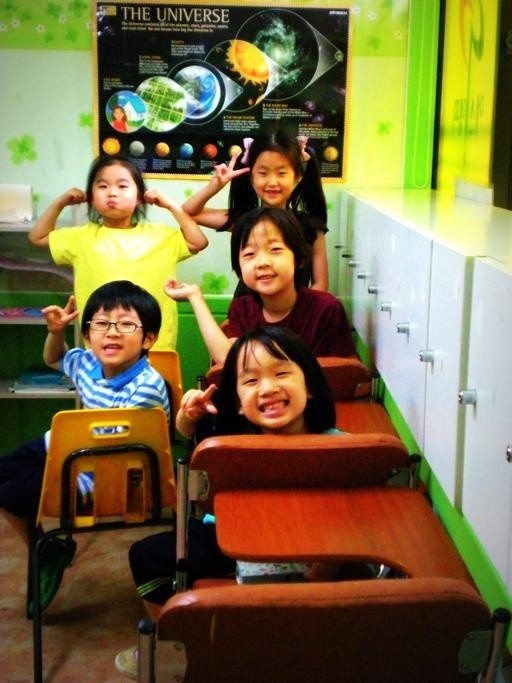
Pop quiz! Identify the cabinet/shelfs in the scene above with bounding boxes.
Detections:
[0,216,83,461]
[336,184,510,592]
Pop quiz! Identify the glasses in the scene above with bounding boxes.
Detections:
[83,319,142,333]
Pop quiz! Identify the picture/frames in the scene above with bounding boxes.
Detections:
[90,0,355,183]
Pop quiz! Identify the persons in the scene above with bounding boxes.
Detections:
[29,154,210,353]
[163,206,358,366]
[181,132,330,299]
[112,322,355,679]
[1,279,173,614]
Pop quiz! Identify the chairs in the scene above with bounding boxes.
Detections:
[26,405,174,681]
[138,484,512,683]
[173,434,422,592]
[194,358,383,406]
[74,347,181,460]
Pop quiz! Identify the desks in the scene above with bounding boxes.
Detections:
[332,395,402,438]
[212,483,478,590]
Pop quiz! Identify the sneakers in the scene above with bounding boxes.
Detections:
[115,645,138,676]
[26,538,76,617]
